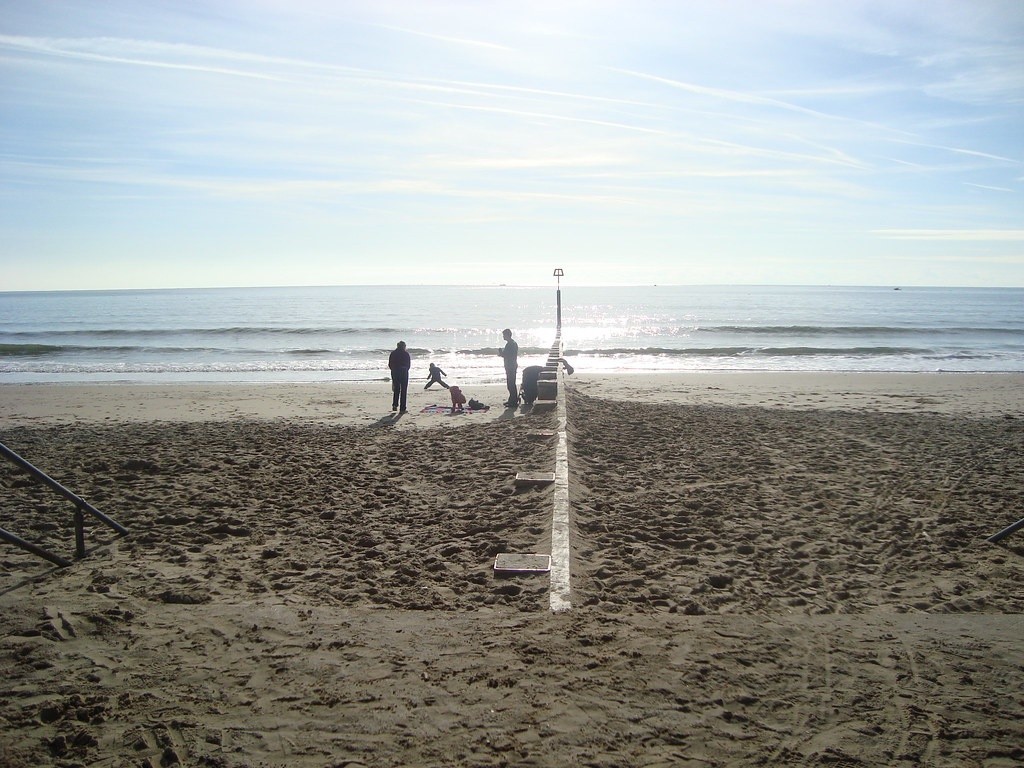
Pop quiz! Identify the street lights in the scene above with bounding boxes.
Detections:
[553,268,564,338]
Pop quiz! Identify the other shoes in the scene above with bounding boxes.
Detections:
[392,407,397,411]
[399,410,408,414]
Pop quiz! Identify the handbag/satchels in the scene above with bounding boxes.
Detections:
[468,399,484,410]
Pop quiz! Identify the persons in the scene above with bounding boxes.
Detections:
[388,341,410,413]
[499,329,519,407]
[424,363,450,390]
[450,386,466,412]
